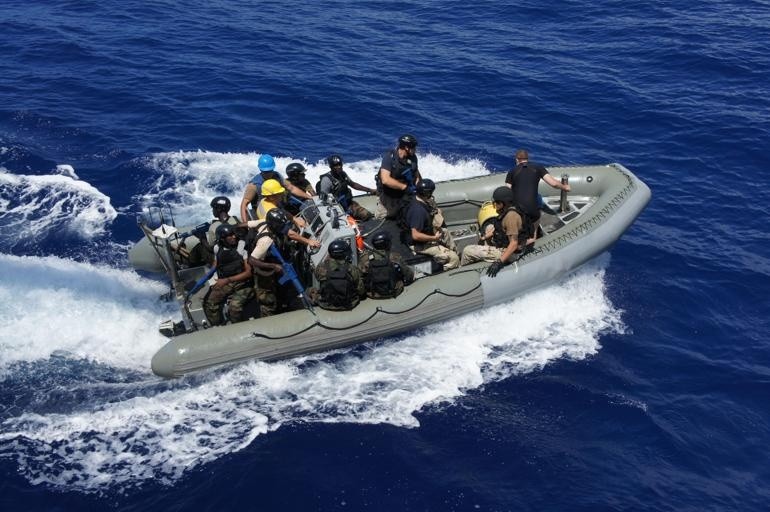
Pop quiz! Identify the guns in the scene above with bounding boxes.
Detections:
[167,220,217,242]
[270,244,317,316]
[284,192,306,210]
[183,266,219,302]
[403,168,418,191]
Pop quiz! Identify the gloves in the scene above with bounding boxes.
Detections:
[486,259,504,277]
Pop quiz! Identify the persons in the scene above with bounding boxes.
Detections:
[502,150,572,230]
[378,134,423,221]
[202,154,413,326]
[403,179,461,272]
[460,185,536,278]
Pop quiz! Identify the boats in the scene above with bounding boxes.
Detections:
[128,164,651,379]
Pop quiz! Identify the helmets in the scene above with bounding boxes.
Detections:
[216,224,235,239]
[399,134,417,148]
[492,186,514,215]
[258,154,307,237]
[328,240,351,259]
[328,155,343,167]
[416,178,435,196]
[211,196,231,212]
[371,231,393,250]
